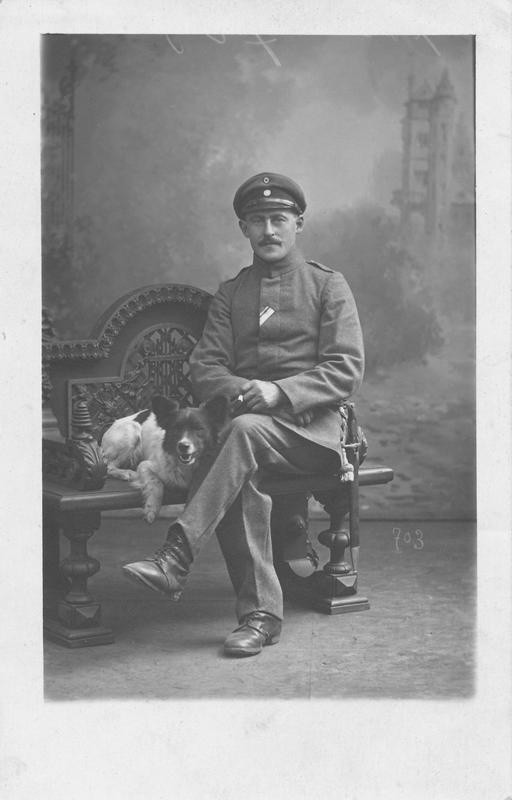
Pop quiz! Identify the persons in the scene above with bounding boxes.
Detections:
[121,172,366,654]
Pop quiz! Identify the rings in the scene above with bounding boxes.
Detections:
[237,394,244,404]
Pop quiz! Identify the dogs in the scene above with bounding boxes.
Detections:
[93,389,230,524]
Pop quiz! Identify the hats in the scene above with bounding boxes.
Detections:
[233,172,306,219]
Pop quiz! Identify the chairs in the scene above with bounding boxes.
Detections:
[42,283,394,648]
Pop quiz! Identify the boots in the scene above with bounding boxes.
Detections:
[120,527,192,603]
[224,611,282,656]
